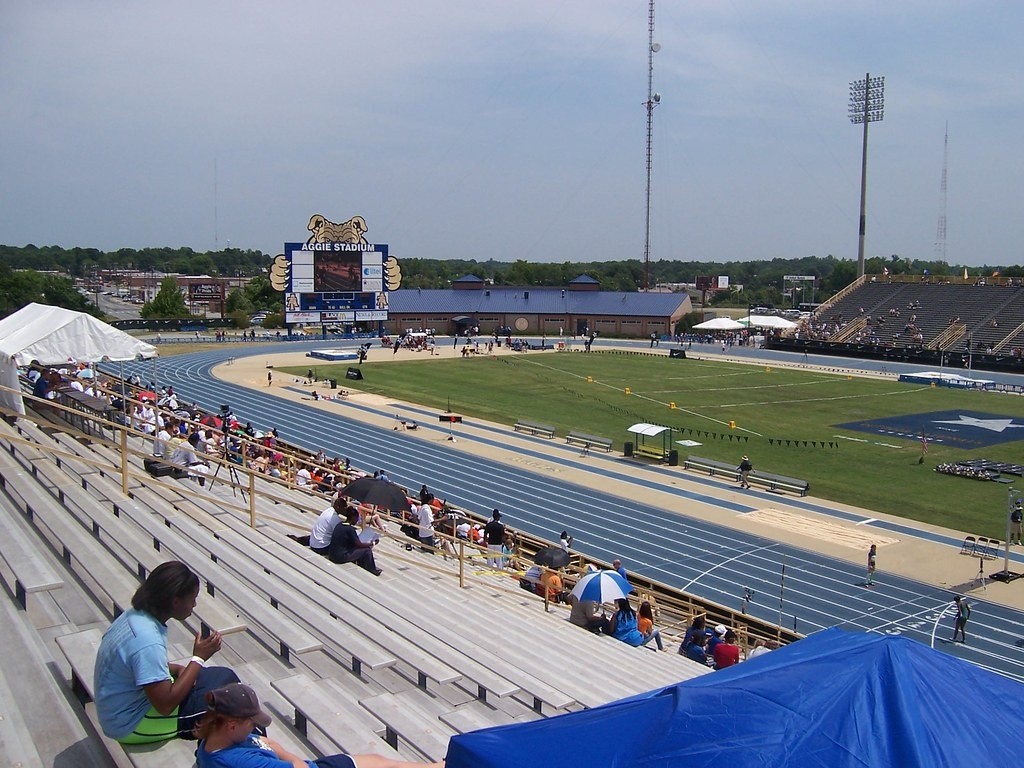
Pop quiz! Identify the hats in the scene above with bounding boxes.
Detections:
[715,624,726,634]
[742,456,749,460]
[189,433,201,441]
[492,509,502,518]
[207,683,272,727]
[1015,504,1023,510]
[272,453,286,463]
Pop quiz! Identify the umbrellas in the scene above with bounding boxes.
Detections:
[536,547,570,568]
[341,474,409,527]
[568,570,632,605]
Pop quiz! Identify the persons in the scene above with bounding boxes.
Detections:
[194,683,445,768]
[1010,506,1024,546]
[542,326,592,350]
[936,315,1024,368]
[794,301,924,345]
[195,329,320,343]
[524,530,779,671]
[27,358,527,576]
[351,326,435,357]
[675,327,788,354]
[308,368,314,386]
[94,561,267,744]
[463,323,528,357]
[869,275,1022,287]
[865,544,876,588]
[650,331,658,348]
[267,370,272,387]
[407,419,421,431]
[949,596,970,643]
[737,455,755,489]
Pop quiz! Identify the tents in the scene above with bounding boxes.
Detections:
[0,302,159,453]
[692,316,797,329]
[448,626,1024,768]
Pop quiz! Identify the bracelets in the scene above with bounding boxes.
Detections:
[189,655,206,667]
[371,538,377,545]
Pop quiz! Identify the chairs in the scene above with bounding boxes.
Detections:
[984,540,1000,561]
[972,537,988,559]
[960,536,977,556]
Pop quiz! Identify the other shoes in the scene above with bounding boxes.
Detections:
[1010,540,1015,546]
[1018,540,1022,546]
[373,569,382,575]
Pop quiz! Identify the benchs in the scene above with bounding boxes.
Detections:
[740,468,810,497]
[0,362,748,768]
